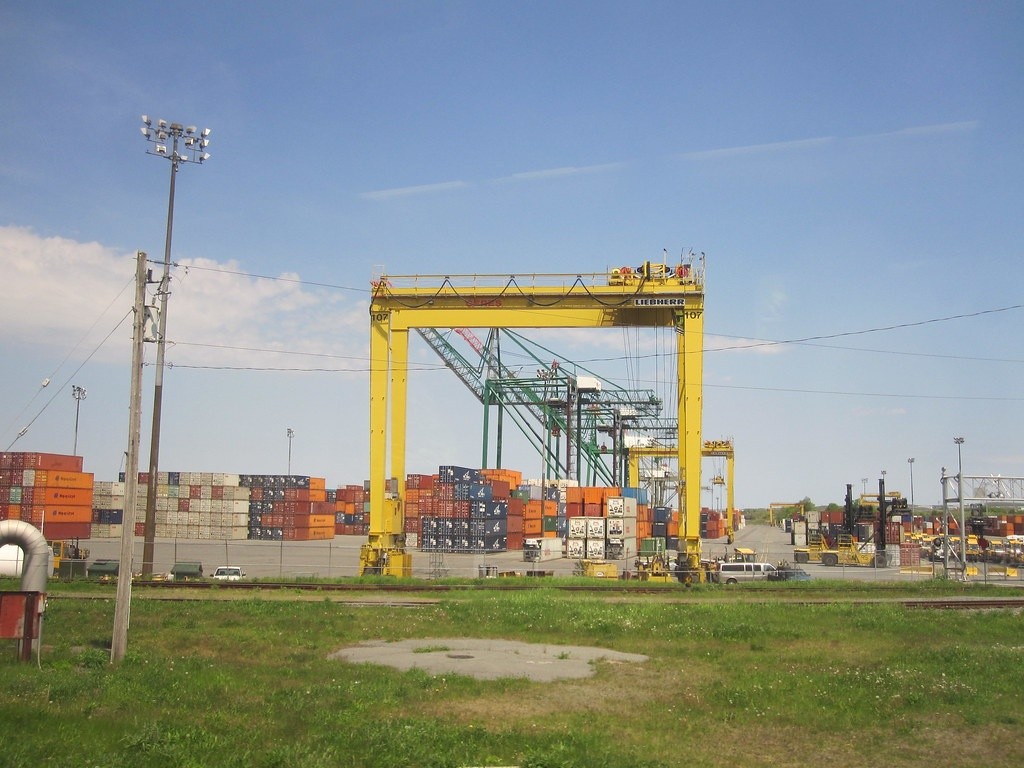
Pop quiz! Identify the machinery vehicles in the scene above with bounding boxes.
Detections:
[369,273,679,505]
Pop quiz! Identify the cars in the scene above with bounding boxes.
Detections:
[207,566,247,583]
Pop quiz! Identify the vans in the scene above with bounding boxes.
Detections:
[716,561,780,585]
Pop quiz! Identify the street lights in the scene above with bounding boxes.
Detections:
[285,428,295,476]
[907,458,916,533]
[881,470,886,480]
[71,383,88,456]
[953,436,966,473]
[140,112,212,578]
[861,477,869,501]
